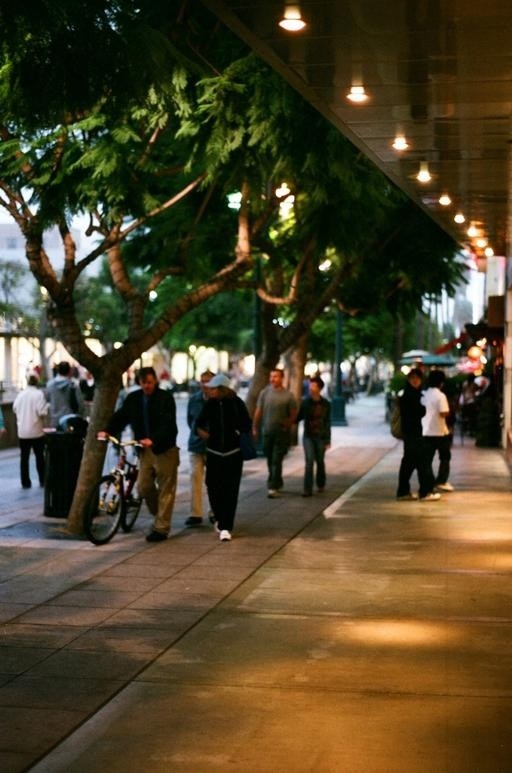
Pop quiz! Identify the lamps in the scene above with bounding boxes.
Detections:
[276,0,308,32]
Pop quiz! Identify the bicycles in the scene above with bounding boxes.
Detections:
[82,432,147,544]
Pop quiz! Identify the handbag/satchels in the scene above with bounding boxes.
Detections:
[391,407,402,438]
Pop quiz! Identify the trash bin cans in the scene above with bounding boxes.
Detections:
[43,433,85,518]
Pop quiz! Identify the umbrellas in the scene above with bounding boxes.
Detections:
[395,353,456,369]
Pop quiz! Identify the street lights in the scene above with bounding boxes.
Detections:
[320,255,353,425]
[229,183,297,455]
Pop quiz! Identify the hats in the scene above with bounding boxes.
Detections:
[205,374,231,387]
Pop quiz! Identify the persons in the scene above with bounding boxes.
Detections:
[291,373,332,496]
[11,372,50,488]
[44,361,85,430]
[461,372,483,439]
[96,366,180,542]
[184,369,215,526]
[251,367,297,497]
[392,367,441,501]
[54,360,176,413]
[418,367,457,491]
[192,373,254,542]
